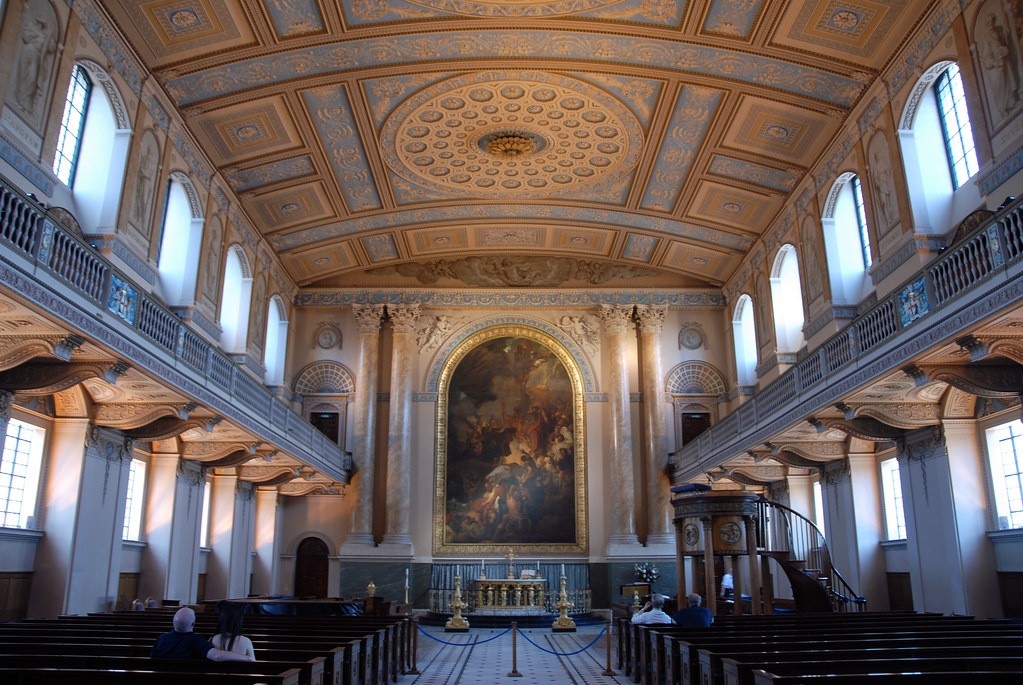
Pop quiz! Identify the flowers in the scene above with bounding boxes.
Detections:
[634,562,659,585]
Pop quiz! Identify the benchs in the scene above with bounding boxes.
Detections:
[616,608,1022,684]
[0,608,415,685]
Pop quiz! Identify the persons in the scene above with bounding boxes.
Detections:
[150,607,255,662]
[721,568,735,613]
[207,606,256,660]
[631,593,672,625]
[670,593,715,627]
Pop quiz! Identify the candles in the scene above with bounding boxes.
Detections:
[405,569,408,587]
[456,565,460,576]
[537,561,540,570]
[561,564,564,577]
[482,560,485,569]
